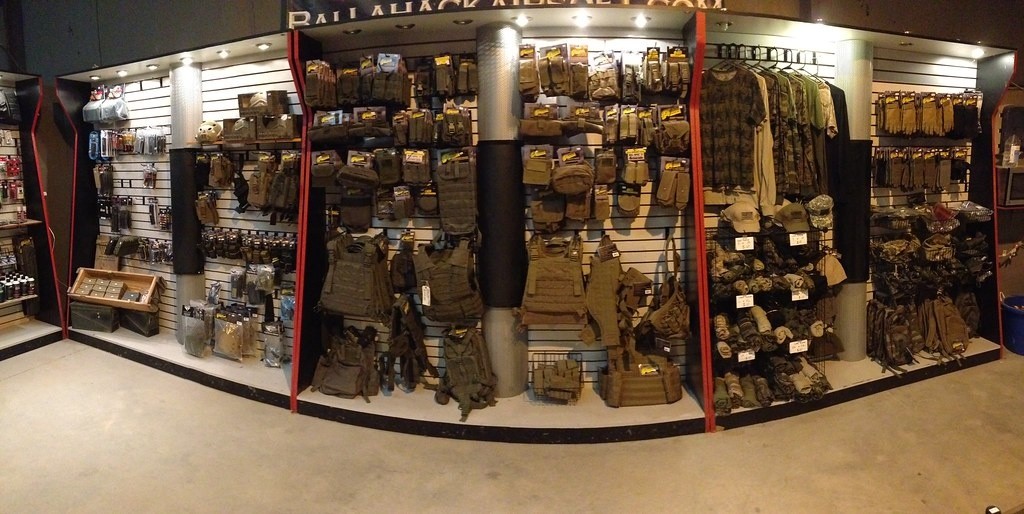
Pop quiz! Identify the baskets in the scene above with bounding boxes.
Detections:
[531,352,582,403]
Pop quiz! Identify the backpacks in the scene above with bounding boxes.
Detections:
[311,326,380,399]
[866,289,980,368]
[441,327,497,413]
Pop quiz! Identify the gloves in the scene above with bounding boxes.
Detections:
[872,88,983,193]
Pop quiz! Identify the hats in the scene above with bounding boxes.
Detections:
[805,195,834,229]
[723,200,761,233]
[774,203,809,233]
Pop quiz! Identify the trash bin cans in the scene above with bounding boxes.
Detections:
[1001,295,1024,356]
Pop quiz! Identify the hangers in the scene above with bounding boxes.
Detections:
[705,42,824,81]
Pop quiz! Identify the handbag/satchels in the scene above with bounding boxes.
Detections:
[551,163,595,195]
[531,199,565,236]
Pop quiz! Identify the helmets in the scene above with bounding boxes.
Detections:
[231,118,250,138]
[249,92,267,114]
[195,121,222,144]
[267,118,287,137]
